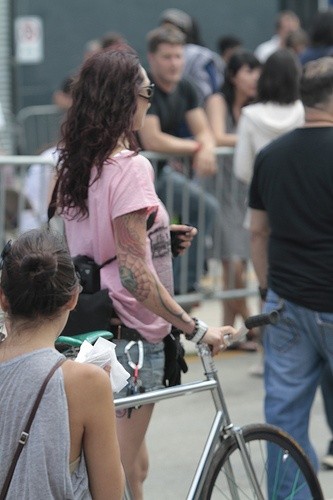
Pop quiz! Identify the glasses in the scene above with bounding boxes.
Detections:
[136,83,156,102]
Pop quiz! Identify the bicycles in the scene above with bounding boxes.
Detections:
[56,311,325,500]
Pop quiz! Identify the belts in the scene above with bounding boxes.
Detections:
[107,323,143,342]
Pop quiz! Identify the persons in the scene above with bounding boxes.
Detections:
[51,7,333,352]
[55,45,237,500]
[247,57,333,500]
[0,230,125,500]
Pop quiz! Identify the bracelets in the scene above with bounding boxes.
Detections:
[259,286,267,301]
[185,318,209,345]
